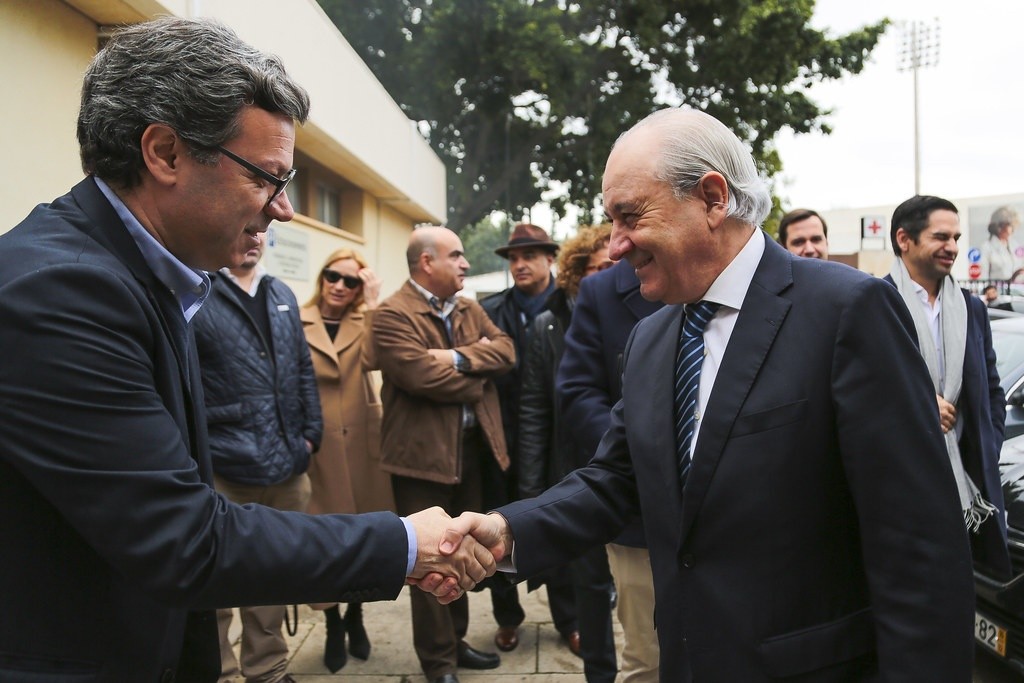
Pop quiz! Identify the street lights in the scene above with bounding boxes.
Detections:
[896,14,940,198]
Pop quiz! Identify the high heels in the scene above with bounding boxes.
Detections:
[322,607,348,675]
[343,607,372,662]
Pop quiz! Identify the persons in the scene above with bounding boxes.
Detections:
[882,196,1010,560]
[479,224,581,653]
[517,223,669,683]
[780,209,830,259]
[195,233,324,683]
[405,108,974,683]
[983,286,998,295]
[298,250,398,672]
[0,18,496,683]
[372,227,515,683]
[981,206,1018,277]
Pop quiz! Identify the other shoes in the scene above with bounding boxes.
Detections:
[611,590,618,609]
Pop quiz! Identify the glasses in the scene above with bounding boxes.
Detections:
[204,141,296,206]
[322,267,363,289]
[580,261,615,274]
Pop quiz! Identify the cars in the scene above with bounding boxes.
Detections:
[971,293,1024,679]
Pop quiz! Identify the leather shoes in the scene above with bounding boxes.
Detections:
[456,643,500,670]
[495,624,518,652]
[434,672,459,683]
[567,629,584,656]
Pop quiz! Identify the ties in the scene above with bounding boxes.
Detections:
[676,300,721,499]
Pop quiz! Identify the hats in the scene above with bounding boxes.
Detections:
[495,223,559,260]
